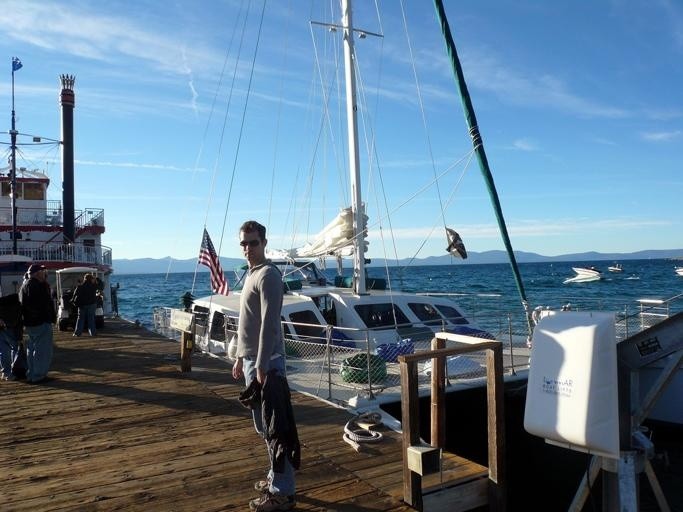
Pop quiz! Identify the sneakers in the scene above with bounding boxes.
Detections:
[32,376,55,385]
[253,479,271,492]
[249,493,296,510]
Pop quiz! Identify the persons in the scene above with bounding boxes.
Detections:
[19,261,55,383]
[95,277,104,292]
[0,292,26,380]
[72,274,104,337]
[230,220,303,512]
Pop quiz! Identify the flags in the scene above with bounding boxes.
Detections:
[197,227,228,296]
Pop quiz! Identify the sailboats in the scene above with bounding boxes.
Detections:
[150,1,557,478]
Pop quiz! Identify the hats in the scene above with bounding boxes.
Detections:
[27,264,46,273]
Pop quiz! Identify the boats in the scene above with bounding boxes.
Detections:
[525,293,681,489]
[571,265,601,276]
[673,267,683,275]
[608,265,623,271]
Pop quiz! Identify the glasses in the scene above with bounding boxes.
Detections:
[240,240,260,246]
